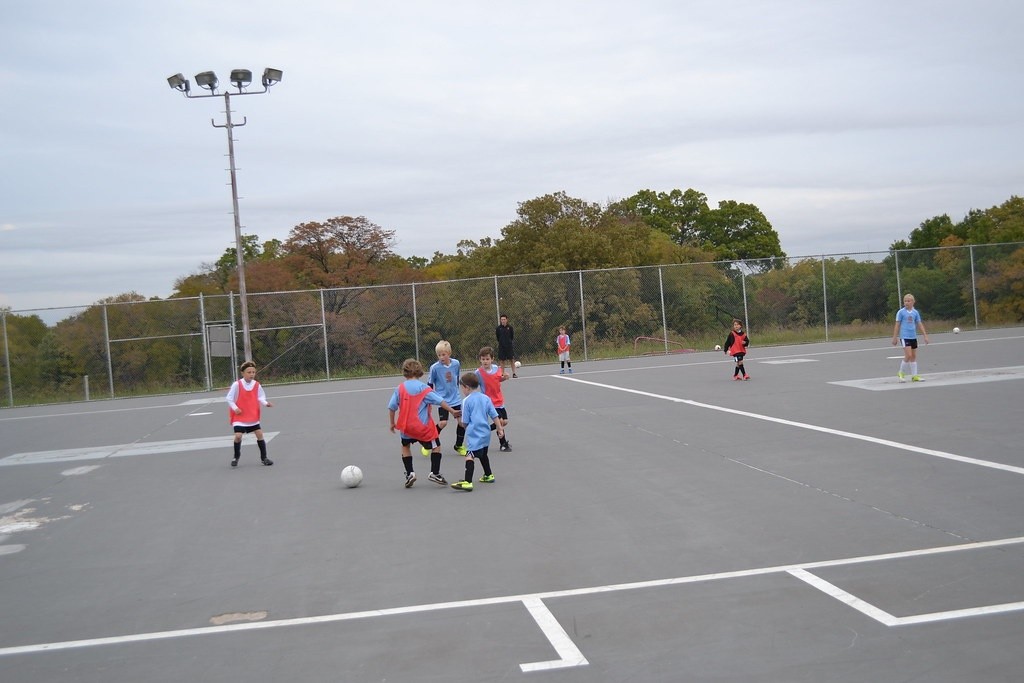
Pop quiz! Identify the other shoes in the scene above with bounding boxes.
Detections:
[569,369,572,374]
[733,375,742,380]
[560,370,565,374]
[743,374,750,380]
[513,374,517,378]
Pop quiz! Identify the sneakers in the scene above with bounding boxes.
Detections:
[500,441,512,452]
[422,446,430,457]
[479,474,494,482]
[912,375,925,382]
[405,472,416,488]
[231,457,239,466]
[897,371,905,383]
[454,445,467,456]
[262,458,273,465]
[451,480,473,492]
[428,471,448,485]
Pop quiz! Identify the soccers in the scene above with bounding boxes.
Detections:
[515,361,521,368]
[953,327,959,334]
[340,465,363,488]
[715,345,721,351]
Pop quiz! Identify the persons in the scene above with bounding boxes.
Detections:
[892,294,928,381]
[451,372,503,492]
[474,347,511,452]
[388,359,460,488]
[496,315,517,378]
[421,340,467,457]
[724,319,750,380]
[557,326,573,374]
[227,361,273,465]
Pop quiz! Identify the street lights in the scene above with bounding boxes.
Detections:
[167,68,283,363]
